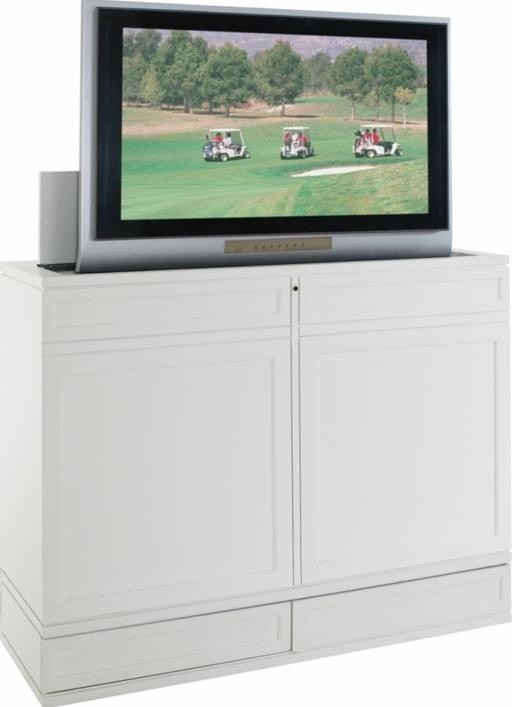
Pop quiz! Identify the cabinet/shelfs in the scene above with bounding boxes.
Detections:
[0,251,512,707]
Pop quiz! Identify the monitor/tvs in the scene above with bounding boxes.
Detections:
[77,0,451,269]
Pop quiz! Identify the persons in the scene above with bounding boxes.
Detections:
[213,133,237,152]
[284,132,308,146]
[362,128,379,145]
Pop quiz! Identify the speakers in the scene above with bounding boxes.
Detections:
[35,170,79,268]
